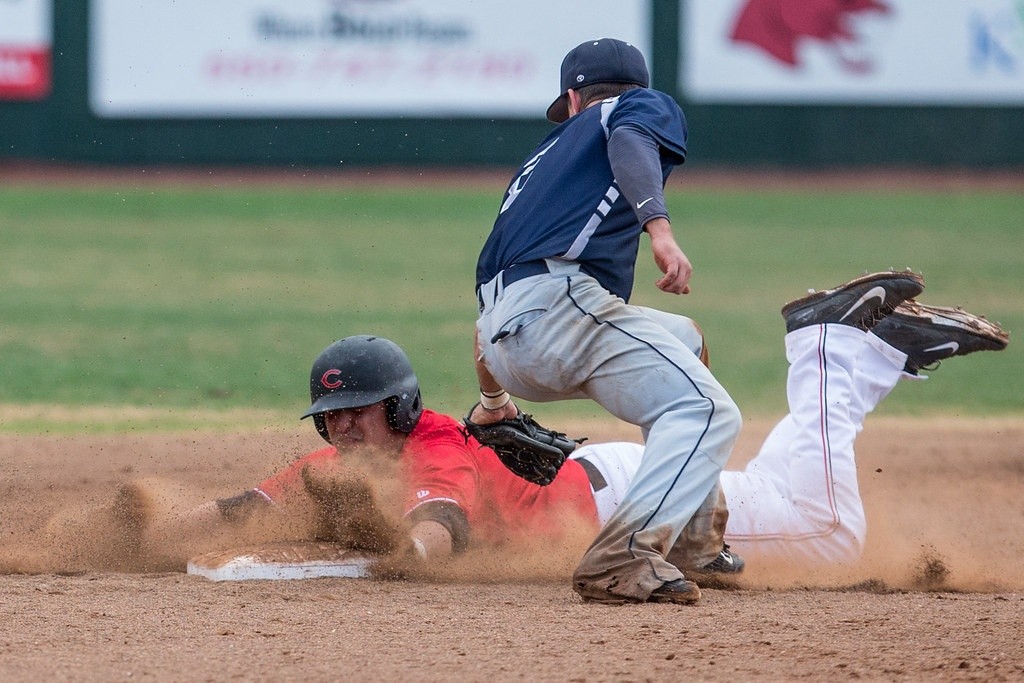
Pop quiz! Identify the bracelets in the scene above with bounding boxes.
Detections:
[478,388,511,411]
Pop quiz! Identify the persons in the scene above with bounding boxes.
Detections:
[140,269,1010,576]
[467,39,746,606]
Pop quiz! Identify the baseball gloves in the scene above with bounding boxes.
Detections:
[463,399,577,488]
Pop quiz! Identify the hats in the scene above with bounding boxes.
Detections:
[546,38,649,124]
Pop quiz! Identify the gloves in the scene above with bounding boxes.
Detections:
[491,325,521,344]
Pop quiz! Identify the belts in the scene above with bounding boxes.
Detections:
[479,258,594,307]
[574,457,609,493]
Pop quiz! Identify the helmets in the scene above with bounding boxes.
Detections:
[300,335,423,447]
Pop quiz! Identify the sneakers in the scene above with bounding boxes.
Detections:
[573,571,701,605]
[781,265,925,337]
[869,299,1010,376]
[693,540,746,576]
